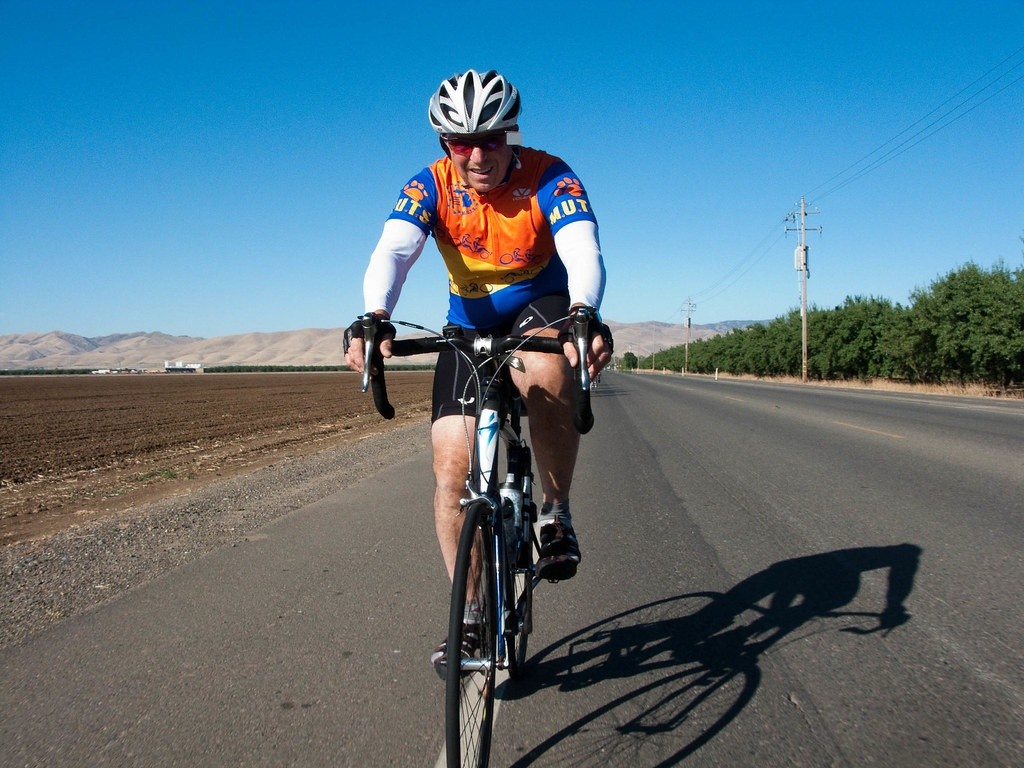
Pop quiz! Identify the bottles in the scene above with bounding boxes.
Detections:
[499,473,522,548]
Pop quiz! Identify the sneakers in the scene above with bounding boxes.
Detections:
[535,513,581,579]
[430,622,480,680]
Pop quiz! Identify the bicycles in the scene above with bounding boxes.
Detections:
[359,308,595,767]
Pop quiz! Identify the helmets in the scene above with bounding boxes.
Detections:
[427,68,521,134]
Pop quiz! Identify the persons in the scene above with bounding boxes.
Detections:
[342,69,615,681]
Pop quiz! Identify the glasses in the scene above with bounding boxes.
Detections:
[441,127,515,156]
